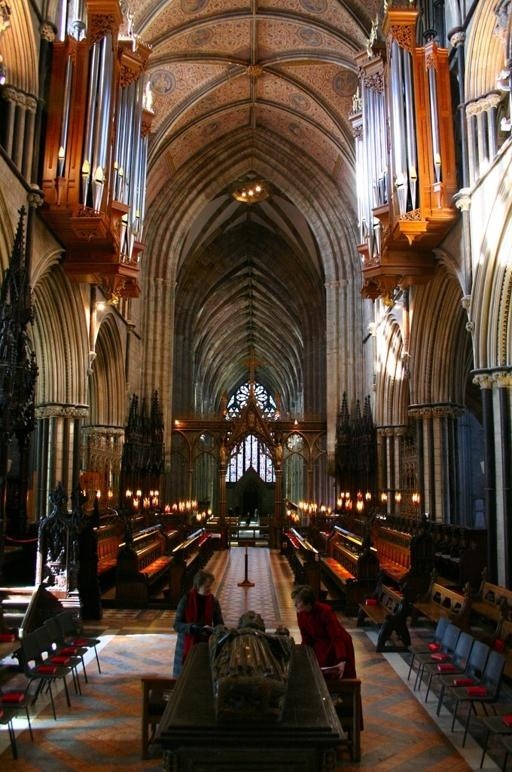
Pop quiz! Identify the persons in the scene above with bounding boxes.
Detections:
[173,570,226,680]
[290,583,365,735]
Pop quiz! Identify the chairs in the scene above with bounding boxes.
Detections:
[0,608,102,762]
[405,615,512,770]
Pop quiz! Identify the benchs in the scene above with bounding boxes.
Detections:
[78,503,227,620]
[280,490,510,658]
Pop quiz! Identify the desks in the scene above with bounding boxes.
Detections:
[138,672,363,764]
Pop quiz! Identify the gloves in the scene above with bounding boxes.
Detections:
[189,624,205,636]
